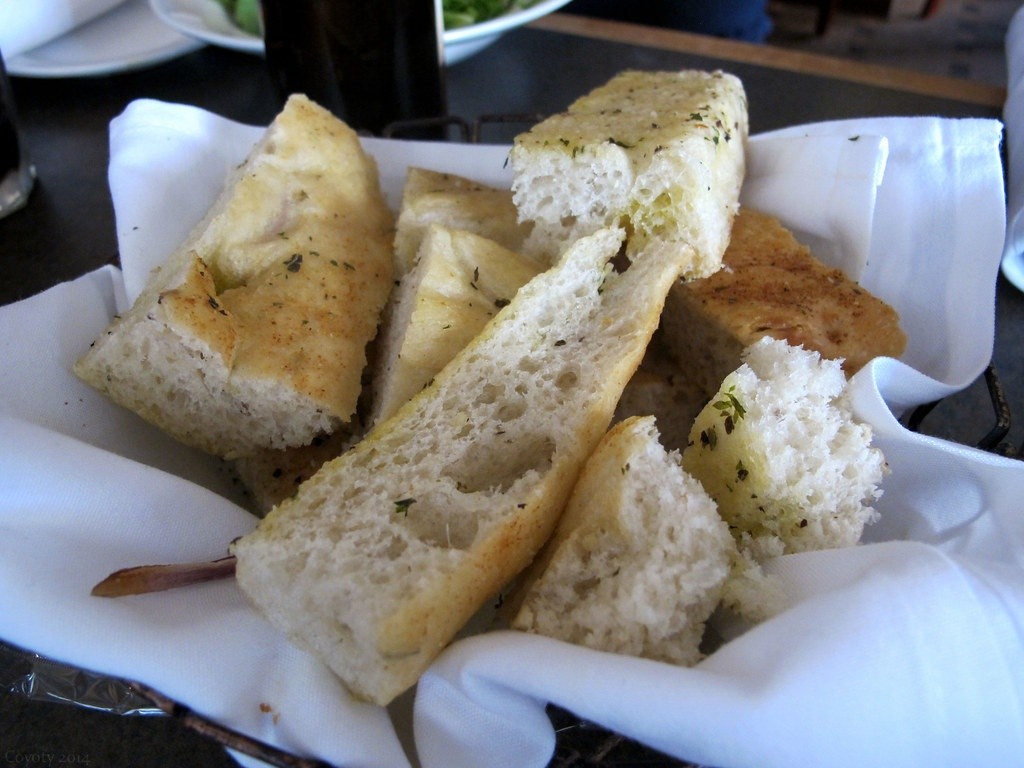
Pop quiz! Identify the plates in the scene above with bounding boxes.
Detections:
[5,1,207,77]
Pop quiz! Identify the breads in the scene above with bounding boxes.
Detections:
[71,71,908,707]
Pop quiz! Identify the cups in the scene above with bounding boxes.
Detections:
[0,53,35,218]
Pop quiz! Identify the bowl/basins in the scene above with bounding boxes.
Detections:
[147,2,573,68]
